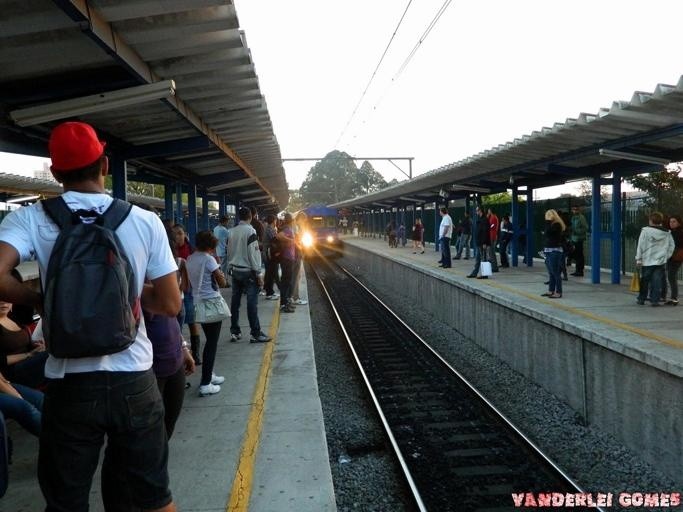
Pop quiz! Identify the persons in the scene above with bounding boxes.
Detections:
[352,218,359,237]
[569,204,588,276]
[635,213,676,306]
[338,216,348,235]
[637,263,668,301]
[664,215,683,306]
[387,205,512,279]
[540,209,568,298]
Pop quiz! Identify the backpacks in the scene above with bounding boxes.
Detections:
[39,195,141,359]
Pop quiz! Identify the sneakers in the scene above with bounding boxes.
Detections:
[258,290,280,300]
[636,298,679,306]
[250,332,271,342]
[541,291,562,298]
[230,333,241,342]
[280,289,307,313]
[200,383,220,395]
[212,375,224,384]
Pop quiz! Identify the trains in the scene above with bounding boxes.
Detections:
[291,206,340,260]
[0,170,234,229]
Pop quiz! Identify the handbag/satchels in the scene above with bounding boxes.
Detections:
[195,294,232,324]
[631,272,640,291]
[481,262,493,276]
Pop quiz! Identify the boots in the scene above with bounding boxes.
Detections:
[190,335,202,365]
